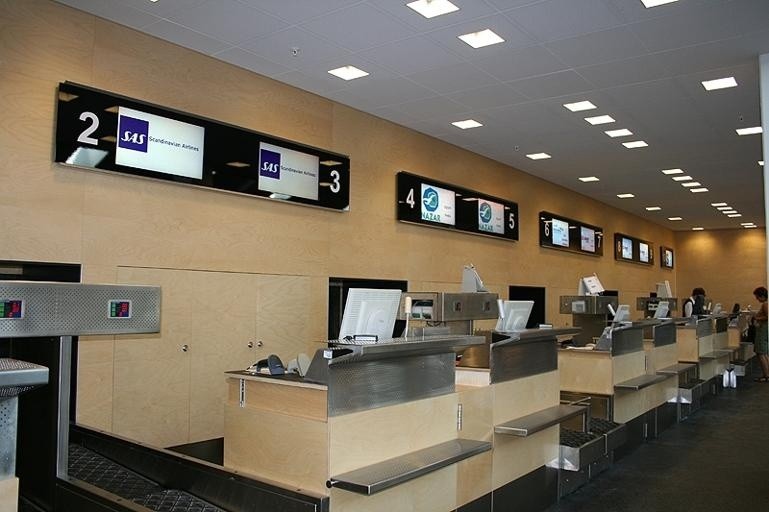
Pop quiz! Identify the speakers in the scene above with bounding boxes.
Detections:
[268,354,284,375]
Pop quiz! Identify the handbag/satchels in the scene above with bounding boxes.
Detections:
[747,316,756,344]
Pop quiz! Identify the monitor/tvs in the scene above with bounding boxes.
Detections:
[712,303,722,315]
[692,295,704,314]
[496,300,535,335]
[653,301,670,319]
[613,305,632,322]
[340,287,402,342]
[730,302,740,321]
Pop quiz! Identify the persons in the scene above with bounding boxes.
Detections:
[753,287,769,382]
[683,288,706,317]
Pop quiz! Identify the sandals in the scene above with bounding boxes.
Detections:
[753,376,769,383]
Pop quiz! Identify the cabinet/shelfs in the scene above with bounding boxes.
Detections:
[224,311,750,512]
